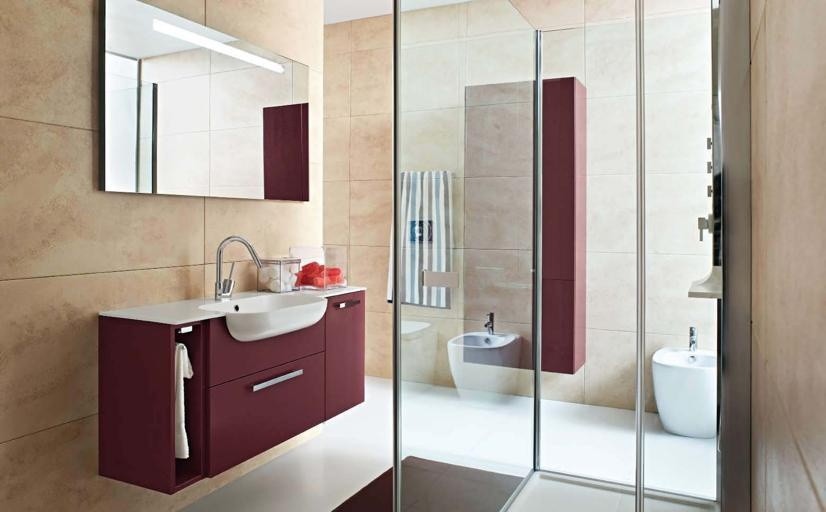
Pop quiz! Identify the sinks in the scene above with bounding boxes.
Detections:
[446,332,523,405]
[197,291,330,342]
[651,346,717,439]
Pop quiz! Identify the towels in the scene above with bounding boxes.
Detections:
[384,169,454,309]
[173,338,196,463]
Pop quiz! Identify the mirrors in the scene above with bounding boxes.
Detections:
[96,0,311,202]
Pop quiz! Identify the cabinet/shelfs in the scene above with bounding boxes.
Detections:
[95,311,207,496]
[324,289,366,424]
[458,73,588,376]
[206,301,325,477]
[260,102,308,203]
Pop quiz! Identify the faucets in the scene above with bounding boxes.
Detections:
[483,312,495,335]
[213,234,263,302]
[688,325,698,351]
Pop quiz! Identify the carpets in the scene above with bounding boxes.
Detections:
[329,455,525,511]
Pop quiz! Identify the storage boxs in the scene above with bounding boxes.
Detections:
[254,258,301,294]
[288,241,348,292]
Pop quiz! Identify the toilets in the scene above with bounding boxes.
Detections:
[401,317,440,398]
